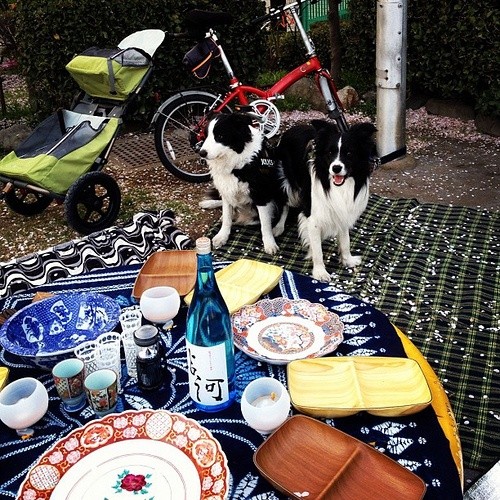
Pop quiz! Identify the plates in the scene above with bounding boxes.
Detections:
[229,297,348,362]
[15,408,232,500]
[184,258,285,314]
[287,356,432,419]
[130,249,198,299]
[252,414,426,500]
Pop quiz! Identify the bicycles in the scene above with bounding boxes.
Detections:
[151,0,350,184]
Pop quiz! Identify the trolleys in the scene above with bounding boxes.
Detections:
[0,28,190,236]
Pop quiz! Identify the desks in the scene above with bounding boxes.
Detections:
[0,262,465,500]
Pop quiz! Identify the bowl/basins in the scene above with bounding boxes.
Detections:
[240,376,291,436]
[0,377,47,430]
[139,286,183,324]
[0,292,121,369]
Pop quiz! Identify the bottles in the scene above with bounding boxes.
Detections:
[133,324,170,398]
[184,237,236,413]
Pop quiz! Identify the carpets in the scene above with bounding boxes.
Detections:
[200,192,500,493]
[0,208,191,296]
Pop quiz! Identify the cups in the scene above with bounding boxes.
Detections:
[51,358,87,412]
[83,369,118,416]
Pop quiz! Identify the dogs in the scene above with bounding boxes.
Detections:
[197,108,288,255]
[276,119,380,281]
